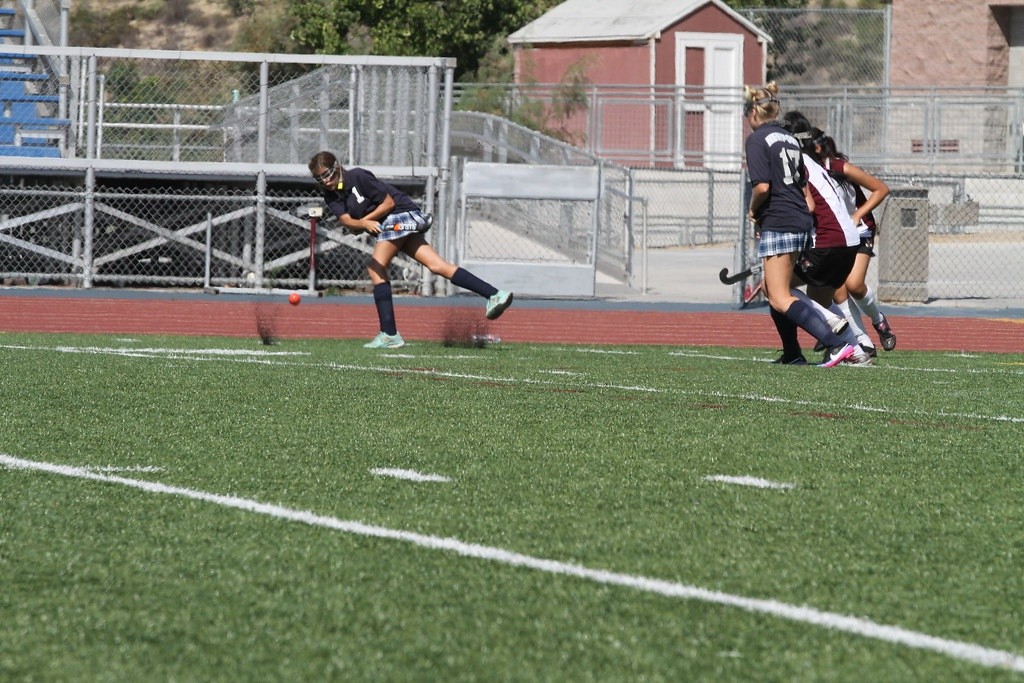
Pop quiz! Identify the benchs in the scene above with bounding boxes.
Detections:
[0,8,71,158]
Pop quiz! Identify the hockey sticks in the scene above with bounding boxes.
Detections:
[720,262,764,285]
[378,213,433,232]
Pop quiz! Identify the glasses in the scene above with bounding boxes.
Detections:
[741,96,780,118]
[311,161,340,186]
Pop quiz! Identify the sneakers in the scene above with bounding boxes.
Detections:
[771,349,807,366]
[363,330,404,347]
[811,316,848,352]
[835,345,873,366]
[858,342,876,358]
[872,311,896,350]
[817,341,855,367]
[485,291,513,320]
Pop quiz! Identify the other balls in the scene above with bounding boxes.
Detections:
[288,293,301,305]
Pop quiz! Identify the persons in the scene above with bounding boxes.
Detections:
[743,81,896,368]
[309,151,513,349]
